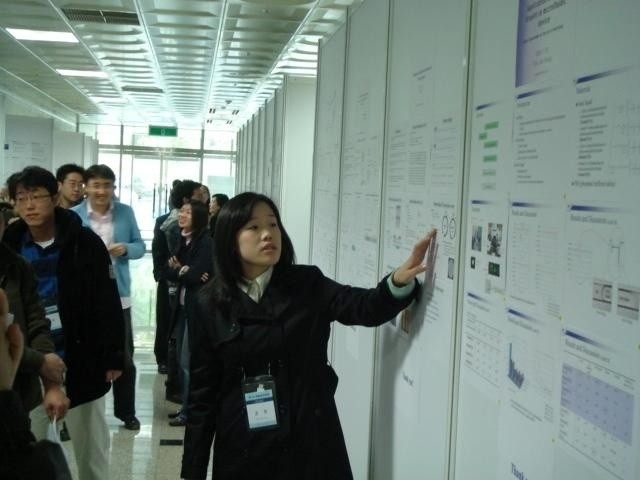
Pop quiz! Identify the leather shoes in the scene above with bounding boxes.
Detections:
[123,415,140,430]
[158,363,168,374]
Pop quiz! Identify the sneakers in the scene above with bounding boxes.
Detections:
[167,408,187,426]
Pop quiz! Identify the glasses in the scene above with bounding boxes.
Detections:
[17,193,51,204]
